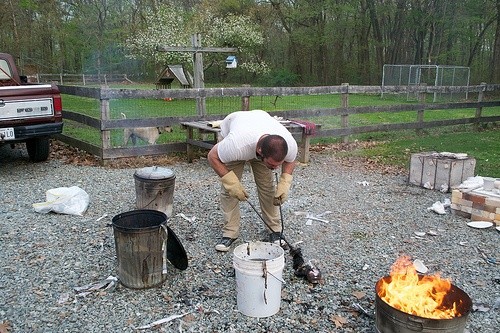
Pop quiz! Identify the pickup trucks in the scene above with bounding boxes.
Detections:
[0,53,64,163]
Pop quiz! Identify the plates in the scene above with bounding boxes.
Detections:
[467,221,493,228]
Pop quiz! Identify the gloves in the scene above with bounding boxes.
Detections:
[220,169,249,202]
[272,172,293,207]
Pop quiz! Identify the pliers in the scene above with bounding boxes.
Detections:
[245,173,292,250]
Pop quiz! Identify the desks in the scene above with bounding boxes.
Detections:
[180,119,322,163]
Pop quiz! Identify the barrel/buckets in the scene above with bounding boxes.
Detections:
[232,240,285,317]
[112,209,169,288]
[377,276,472,333]
[133,167,175,220]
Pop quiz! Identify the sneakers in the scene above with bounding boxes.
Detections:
[270,232,285,246]
[215,237,238,252]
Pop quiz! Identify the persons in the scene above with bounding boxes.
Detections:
[208,110,298,251]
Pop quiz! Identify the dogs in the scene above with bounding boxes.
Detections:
[121,112,173,145]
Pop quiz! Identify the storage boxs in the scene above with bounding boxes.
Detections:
[408,152,476,192]
